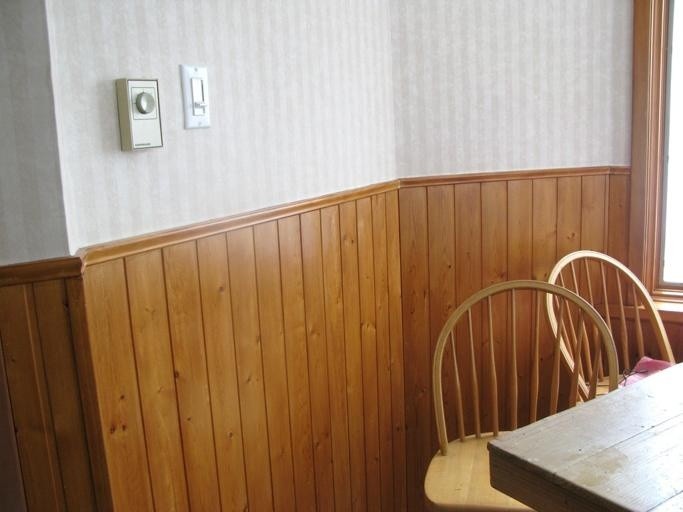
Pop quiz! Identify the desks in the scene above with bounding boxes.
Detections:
[489,360,683,512]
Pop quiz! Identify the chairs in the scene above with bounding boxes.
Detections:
[425,279,619,512]
[543,248,676,404]
